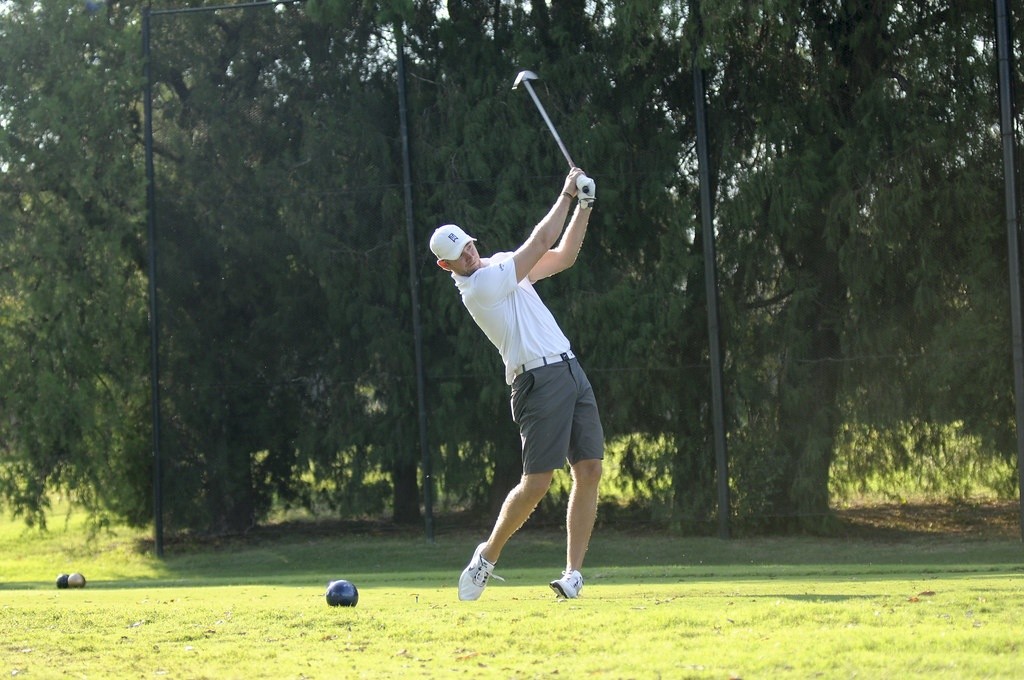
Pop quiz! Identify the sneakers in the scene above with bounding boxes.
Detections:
[549,570,583,599]
[458,542,505,601]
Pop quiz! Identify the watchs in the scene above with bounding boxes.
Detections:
[579,199,594,208]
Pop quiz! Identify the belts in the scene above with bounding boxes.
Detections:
[512,350,575,383]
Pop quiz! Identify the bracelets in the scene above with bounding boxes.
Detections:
[561,192,574,200]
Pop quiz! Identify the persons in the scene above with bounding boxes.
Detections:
[430,168,606,601]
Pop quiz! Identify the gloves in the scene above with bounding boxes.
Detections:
[560,167,586,200]
[577,174,596,207]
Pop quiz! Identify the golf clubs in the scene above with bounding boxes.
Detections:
[512,69,591,194]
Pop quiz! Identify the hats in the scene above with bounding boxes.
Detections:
[429,224,477,260]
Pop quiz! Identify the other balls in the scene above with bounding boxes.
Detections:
[326,579,358,606]
[68,573,85,587]
[56,574,69,588]
